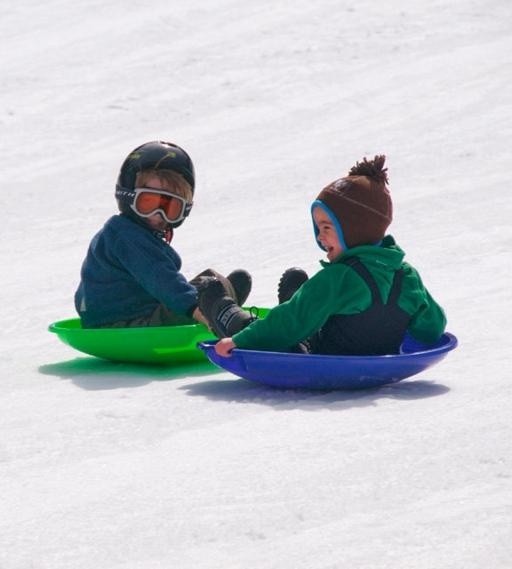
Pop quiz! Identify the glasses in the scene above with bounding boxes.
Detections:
[116,186,194,225]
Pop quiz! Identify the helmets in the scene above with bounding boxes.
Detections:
[117,139,195,227]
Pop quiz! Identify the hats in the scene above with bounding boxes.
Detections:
[311,155,393,250]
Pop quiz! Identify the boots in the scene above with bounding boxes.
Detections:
[198,269,258,336]
[278,267,307,305]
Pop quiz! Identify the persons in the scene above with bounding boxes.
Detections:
[193,151,450,362]
[72,139,253,327]
